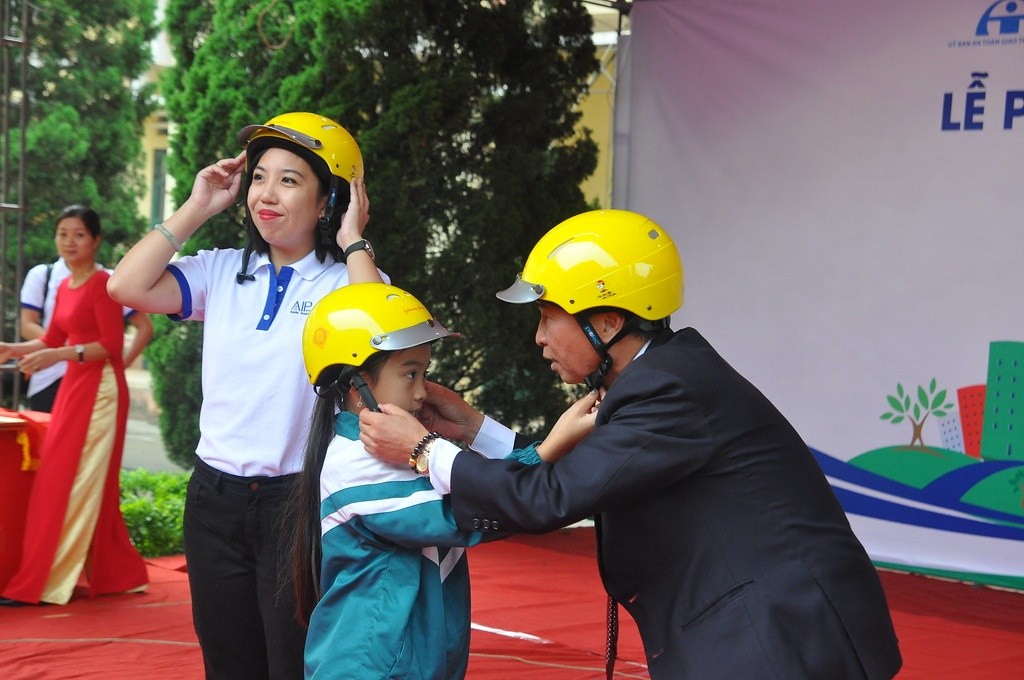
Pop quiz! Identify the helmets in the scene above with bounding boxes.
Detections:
[496,209,683,321]
[239,112,364,207]
[302,282,460,387]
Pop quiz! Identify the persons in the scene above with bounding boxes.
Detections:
[279,284,607,680]
[357,209,902,680]
[106,112,391,680]
[0,204,154,607]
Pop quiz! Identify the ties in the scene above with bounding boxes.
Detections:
[606,596,618,680]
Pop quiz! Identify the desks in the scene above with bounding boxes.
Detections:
[0,408,52,599]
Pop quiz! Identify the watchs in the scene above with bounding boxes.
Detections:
[415,438,441,475]
[343,239,376,265]
[75,344,84,363]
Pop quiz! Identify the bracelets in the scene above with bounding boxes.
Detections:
[154,223,181,252]
[409,431,446,475]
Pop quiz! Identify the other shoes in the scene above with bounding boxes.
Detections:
[0,597,49,606]
